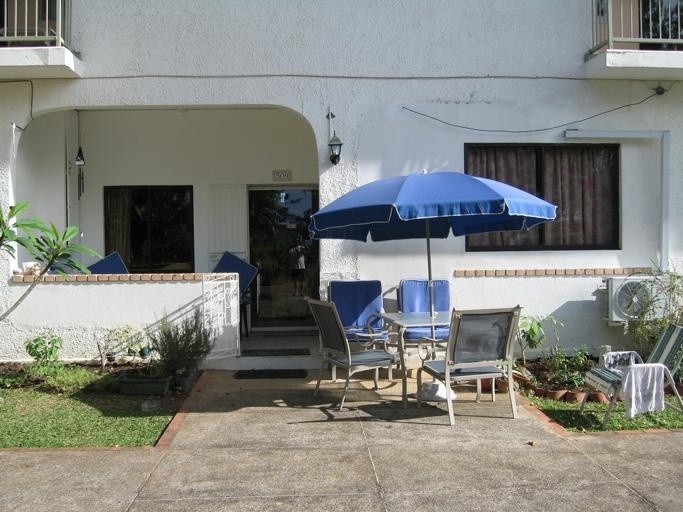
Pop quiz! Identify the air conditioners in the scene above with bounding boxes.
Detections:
[598,274,682,327]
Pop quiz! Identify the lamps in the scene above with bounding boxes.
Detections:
[328,130,345,165]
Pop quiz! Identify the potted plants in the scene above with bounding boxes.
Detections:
[482,314,624,404]
[97,306,217,399]
[621,251,683,397]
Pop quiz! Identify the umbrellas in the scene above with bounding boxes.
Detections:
[308,169,559,382]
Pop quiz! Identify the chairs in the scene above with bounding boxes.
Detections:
[302,278,524,426]
[576,323,682,429]
[240,291,253,341]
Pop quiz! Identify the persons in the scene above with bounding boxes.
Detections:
[286,237,309,296]
